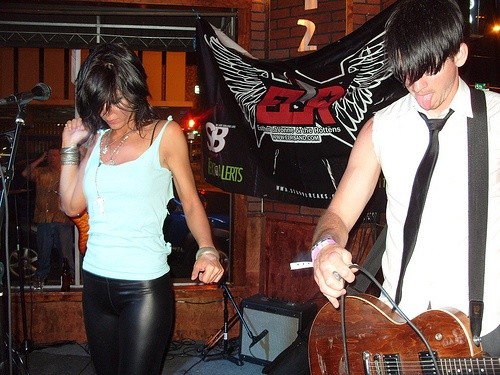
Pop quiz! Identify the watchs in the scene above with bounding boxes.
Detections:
[290,234,335,271]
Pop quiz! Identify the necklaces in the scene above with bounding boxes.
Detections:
[101,128,133,155]
[99,128,135,163]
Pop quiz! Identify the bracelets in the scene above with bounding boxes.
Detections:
[195,247,220,263]
[61,147,80,165]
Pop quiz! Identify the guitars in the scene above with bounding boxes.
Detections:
[308,293,500,375]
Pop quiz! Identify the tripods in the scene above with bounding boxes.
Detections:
[200,192,244,366]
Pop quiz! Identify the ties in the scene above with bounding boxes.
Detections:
[392,108,455,312]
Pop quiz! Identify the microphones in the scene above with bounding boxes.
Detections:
[249,329,269,348]
[0,81,52,106]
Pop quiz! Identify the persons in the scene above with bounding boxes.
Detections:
[58,43,223,375]
[22,148,76,289]
[291,0,500,358]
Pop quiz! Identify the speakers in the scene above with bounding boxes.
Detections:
[238,293,312,364]
